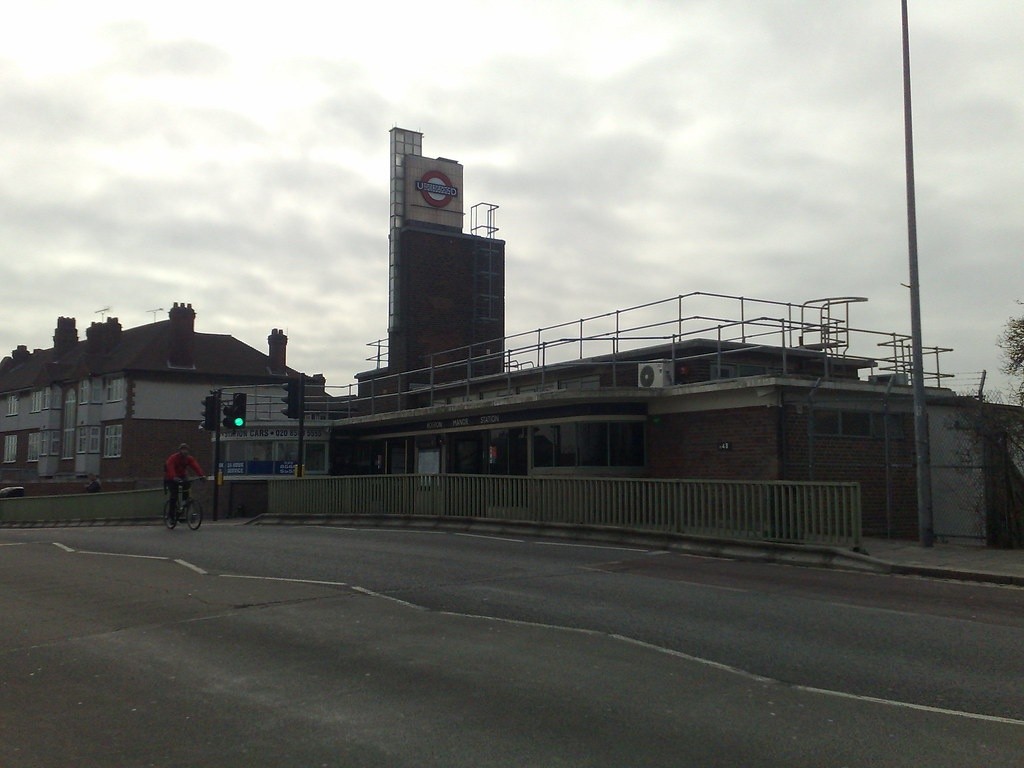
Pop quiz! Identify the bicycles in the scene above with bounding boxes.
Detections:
[164,476,212,530]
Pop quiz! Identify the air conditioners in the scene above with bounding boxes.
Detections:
[637,358,675,388]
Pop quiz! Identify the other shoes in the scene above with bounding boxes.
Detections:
[167,515,174,524]
[181,495,189,500]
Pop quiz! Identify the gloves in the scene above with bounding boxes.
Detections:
[174,476,183,486]
[199,474,207,484]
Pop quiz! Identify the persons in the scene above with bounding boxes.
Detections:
[86,473,100,493]
[165,443,205,525]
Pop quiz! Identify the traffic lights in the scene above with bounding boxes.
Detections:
[281,373,304,419]
[201,389,220,431]
[223,392,246,429]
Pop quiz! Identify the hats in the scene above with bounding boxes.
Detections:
[178,443,191,450]
